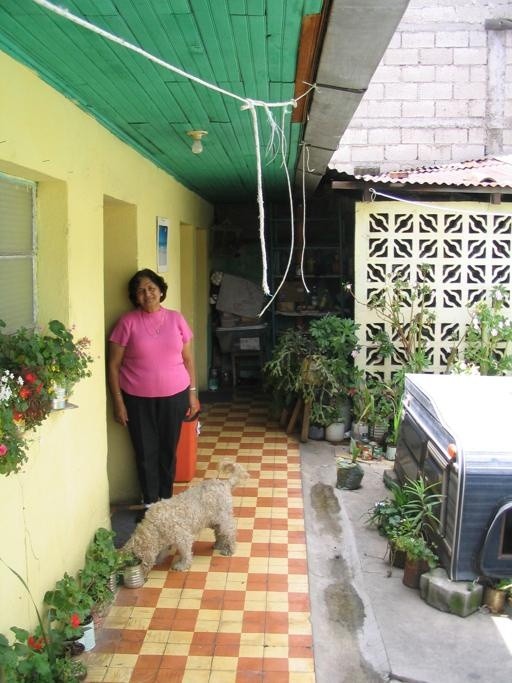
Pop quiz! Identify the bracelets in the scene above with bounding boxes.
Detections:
[187,386,197,391]
[111,390,122,398]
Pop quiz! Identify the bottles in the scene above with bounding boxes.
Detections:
[312,285,329,312]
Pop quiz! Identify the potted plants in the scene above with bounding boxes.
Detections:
[260,312,401,461]
[484,578,512,614]
[358,470,448,591]
[42,528,145,652]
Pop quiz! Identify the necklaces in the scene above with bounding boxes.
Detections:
[141,308,166,336]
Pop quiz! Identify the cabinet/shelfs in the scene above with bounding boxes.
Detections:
[269,196,344,346]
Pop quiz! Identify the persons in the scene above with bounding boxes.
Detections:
[107,267,200,522]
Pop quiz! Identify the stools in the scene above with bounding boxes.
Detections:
[231,351,267,400]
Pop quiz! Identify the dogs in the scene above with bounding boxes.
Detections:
[120,459,248,573]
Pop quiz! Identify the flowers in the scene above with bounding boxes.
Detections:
[0,613,88,682]
[0,317,102,477]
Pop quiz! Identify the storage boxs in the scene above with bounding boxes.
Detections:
[215,322,270,354]
